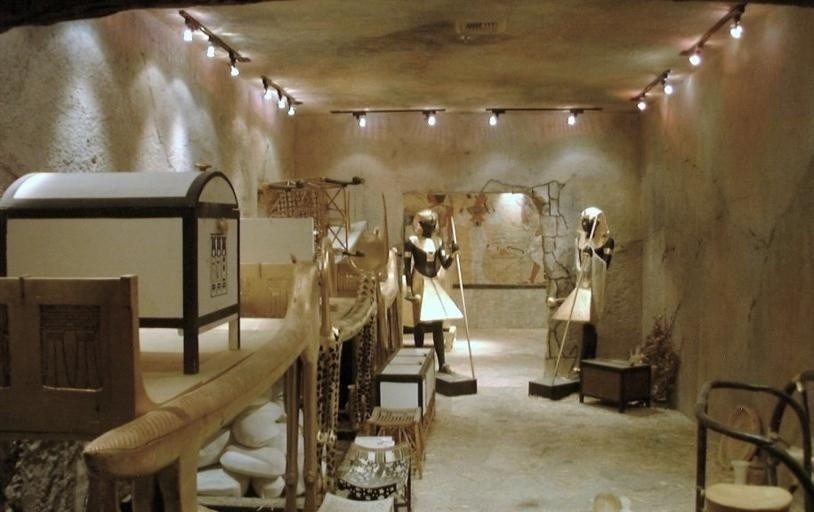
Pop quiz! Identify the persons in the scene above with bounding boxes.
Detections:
[403,208,460,374]
[551,207,615,361]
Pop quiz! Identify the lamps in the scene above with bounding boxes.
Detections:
[634,68,673,112]
[351,110,437,126]
[178,11,252,74]
[488,109,583,125]
[261,76,304,116]
[680,0,744,68]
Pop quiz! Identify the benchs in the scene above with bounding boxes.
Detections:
[580,356,653,414]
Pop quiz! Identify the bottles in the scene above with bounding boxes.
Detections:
[750,455,767,484]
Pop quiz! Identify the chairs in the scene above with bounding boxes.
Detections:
[692,376,814,512]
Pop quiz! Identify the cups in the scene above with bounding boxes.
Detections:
[731,459,748,483]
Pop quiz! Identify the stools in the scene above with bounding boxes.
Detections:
[368,406,427,480]
[335,441,414,512]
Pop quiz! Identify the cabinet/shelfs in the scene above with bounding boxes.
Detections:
[374,345,440,435]
